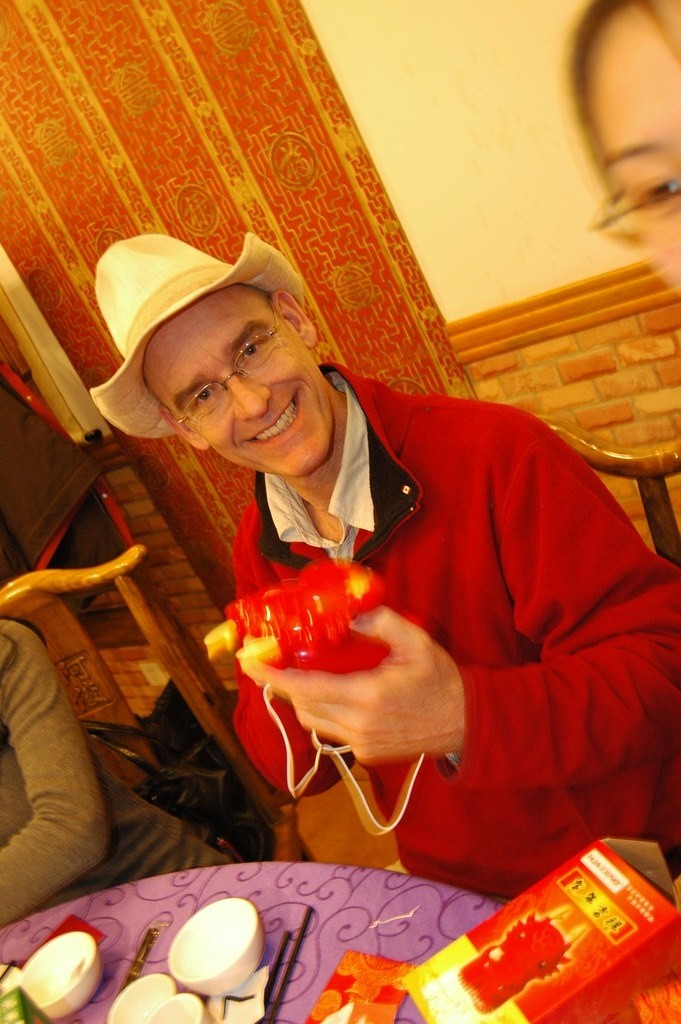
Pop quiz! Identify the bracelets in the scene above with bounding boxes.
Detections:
[441,751,462,767]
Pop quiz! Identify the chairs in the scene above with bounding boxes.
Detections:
[536,416,681,570]
[1,544,302,862]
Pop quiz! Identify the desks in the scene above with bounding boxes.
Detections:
[1,860,509,1024]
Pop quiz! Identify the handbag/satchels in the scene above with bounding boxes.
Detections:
[79,719,272,862]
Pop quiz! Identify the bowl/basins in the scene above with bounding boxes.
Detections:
[16,932,102,1021]
[139,994,216,1022]
[166,897,265,993]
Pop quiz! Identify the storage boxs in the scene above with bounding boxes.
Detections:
[402,838,680,1024]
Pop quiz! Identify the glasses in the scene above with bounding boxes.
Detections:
[170,300,278,424]
[595,187,681,256]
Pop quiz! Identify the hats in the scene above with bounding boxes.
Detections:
[90,231,304,438]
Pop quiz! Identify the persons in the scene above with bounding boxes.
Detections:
[0,617,235,928]
[88,232,681,904]
[569,1,681,306]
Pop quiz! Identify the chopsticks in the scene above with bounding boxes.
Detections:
[253,905,312,1022]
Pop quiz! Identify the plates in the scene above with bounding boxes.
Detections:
[104,973,176,1022]
